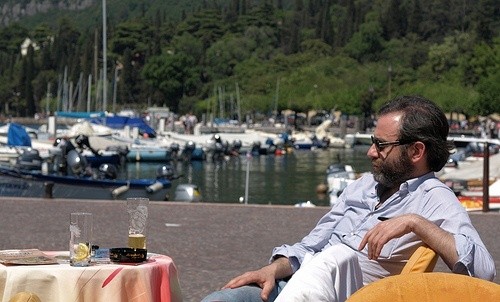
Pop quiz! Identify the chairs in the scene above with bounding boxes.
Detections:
[343,244,500,302]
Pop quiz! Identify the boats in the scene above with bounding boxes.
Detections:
[327,112,500,213]
[0,0,348,188]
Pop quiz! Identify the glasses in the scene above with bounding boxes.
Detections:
[370,135,404,152]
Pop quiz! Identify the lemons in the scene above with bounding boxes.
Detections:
[74,243,89,261]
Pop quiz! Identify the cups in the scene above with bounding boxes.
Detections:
[139,249,147,264]
[109,248,119,262]
[95,249,111,263]
[119,248,140,265]
[69,212,93,267]
[127,197,149,249]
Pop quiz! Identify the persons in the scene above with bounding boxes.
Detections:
[179,111,198,134]
[198,95,496,302]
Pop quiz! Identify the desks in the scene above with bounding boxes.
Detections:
[0,251,184,302]
